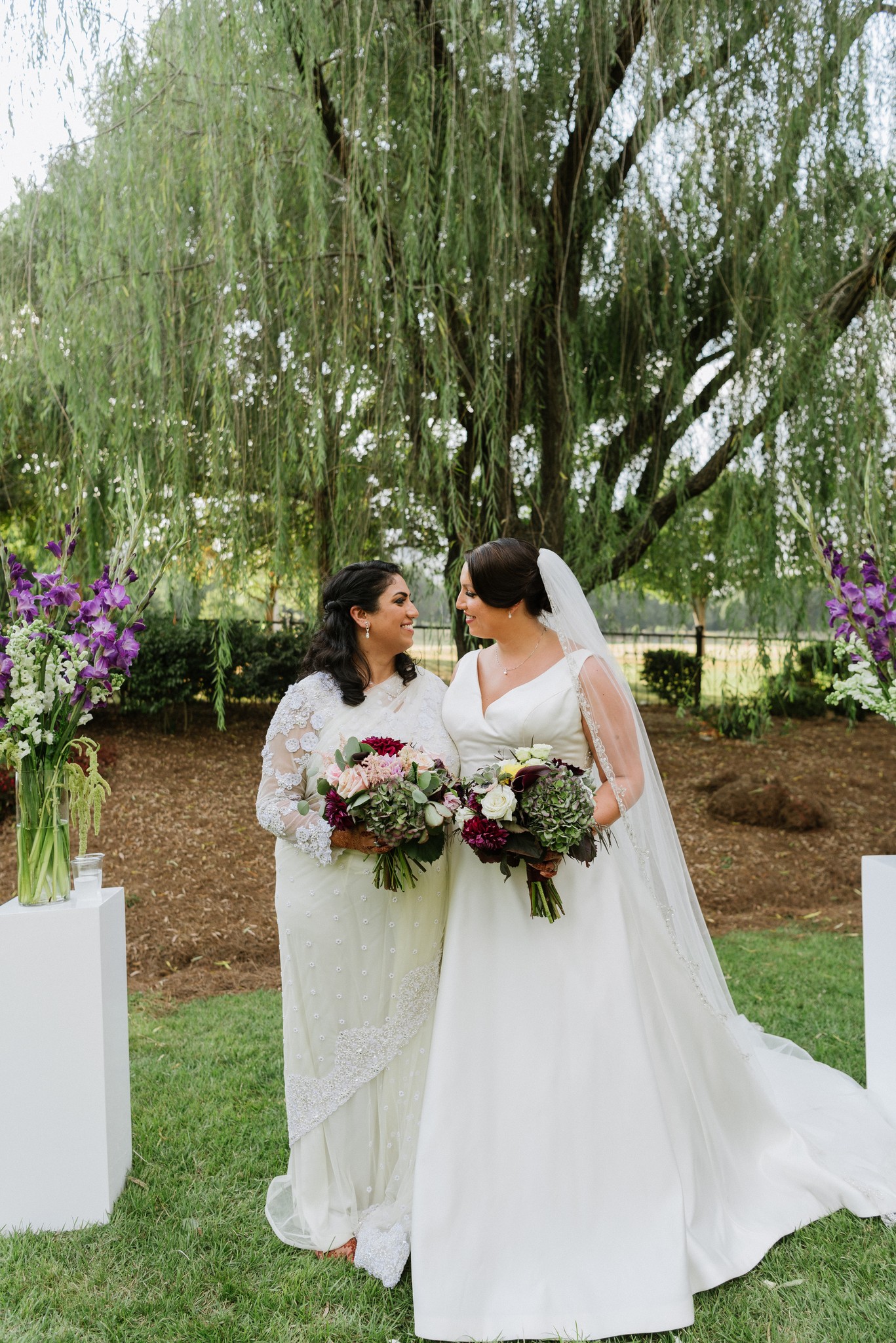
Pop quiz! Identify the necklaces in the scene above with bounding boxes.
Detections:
[495,626,549,675]
[355,667,403,698]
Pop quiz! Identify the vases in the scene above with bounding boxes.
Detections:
[13,766,71,908]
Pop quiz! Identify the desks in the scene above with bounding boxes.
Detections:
[859,851,896,1122]
[0,887,134,1238]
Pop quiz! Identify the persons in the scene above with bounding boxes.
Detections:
[441,536,651,1261]
[253,560,451,1267]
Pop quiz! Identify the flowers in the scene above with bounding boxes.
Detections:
[777,457,896,724]
[4,515,163,908]
[454,737,621,926]
[294,733,463,893]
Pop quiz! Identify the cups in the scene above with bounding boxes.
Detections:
[70,856,100,902]
[76,853,105,893]
